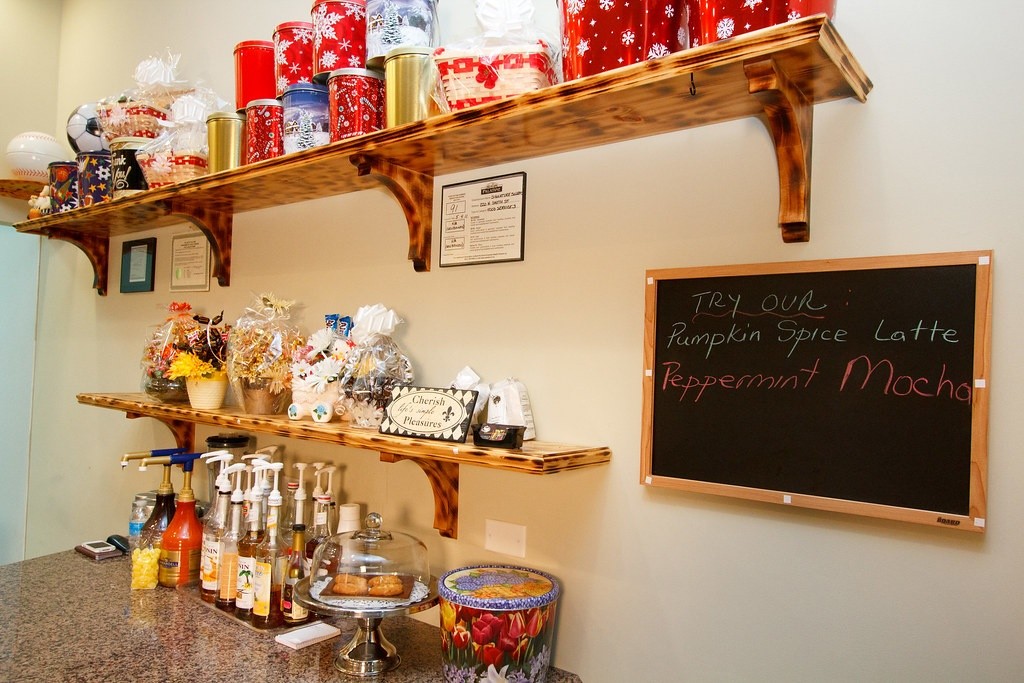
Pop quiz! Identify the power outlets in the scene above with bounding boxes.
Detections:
[482,518,526,559]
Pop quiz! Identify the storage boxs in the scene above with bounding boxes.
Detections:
[471,423,527,449]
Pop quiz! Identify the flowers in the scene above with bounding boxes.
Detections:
[166,349,216,382]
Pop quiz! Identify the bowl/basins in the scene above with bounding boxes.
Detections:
[184,372,227,410]
[232,374,286,415]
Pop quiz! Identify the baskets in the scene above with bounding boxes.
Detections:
[96,101,172,142]
[434,46,558,116]
[137,150,208,191]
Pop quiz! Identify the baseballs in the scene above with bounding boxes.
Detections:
[6,131,65,183]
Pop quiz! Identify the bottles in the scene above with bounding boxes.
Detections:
[129,479,340,627]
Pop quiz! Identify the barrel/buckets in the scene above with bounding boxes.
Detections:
[205,0,441,176]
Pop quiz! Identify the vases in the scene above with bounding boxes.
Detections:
[185,372,229,411]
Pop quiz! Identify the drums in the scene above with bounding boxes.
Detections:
[556,0,838,85]
[437,564,562,683]
[47,136,155,212]
[232,0,439,164]
[205,112,248,175]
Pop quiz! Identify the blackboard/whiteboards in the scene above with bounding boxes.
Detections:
[640,250,995,532]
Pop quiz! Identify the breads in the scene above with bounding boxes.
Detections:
[332,573,403,596]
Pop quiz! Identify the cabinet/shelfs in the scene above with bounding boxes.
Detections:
[0,13,873,543]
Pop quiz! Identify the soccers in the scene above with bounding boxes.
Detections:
[66,101,111,152]
